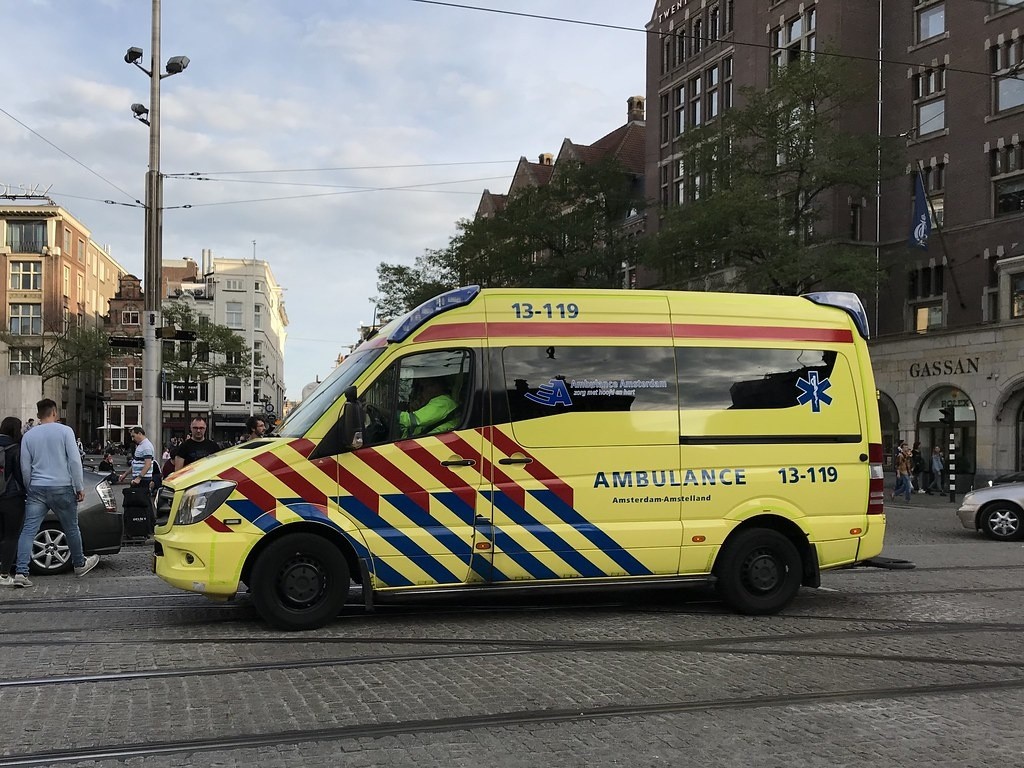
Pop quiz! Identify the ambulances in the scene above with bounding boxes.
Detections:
[171,278,889,636]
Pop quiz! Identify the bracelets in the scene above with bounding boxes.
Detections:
[138,473,143,478]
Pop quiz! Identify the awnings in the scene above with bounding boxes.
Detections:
[215,422,248,426]
[162,422,192,428]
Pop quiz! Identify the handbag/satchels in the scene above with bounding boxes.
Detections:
[121,482,151,508]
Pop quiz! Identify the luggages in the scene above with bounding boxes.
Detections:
[123,484,154,539]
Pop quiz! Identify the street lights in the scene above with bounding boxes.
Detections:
[121,43,192,494]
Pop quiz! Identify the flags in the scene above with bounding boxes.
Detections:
[906,176,931,251]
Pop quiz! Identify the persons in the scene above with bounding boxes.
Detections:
[890,439,948,504]
[0,416,268,584]
[13,398,99,589]
[361,371,465,440]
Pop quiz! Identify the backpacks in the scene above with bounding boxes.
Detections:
[0,443,18,496]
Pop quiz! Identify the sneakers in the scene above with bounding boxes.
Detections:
[74,554,99,577]
[0,575,15,585]
[13,574,33,587]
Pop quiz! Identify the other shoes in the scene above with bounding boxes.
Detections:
[940,493,947,496]
[925,490,934,495]
[903,501,912,505]
[918,489,926,494]
[891,493,896,502]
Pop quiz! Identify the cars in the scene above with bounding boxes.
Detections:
[22,467,124,576]
[960,477,1024,545]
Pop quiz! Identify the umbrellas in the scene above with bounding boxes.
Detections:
[97,423,124,429]
